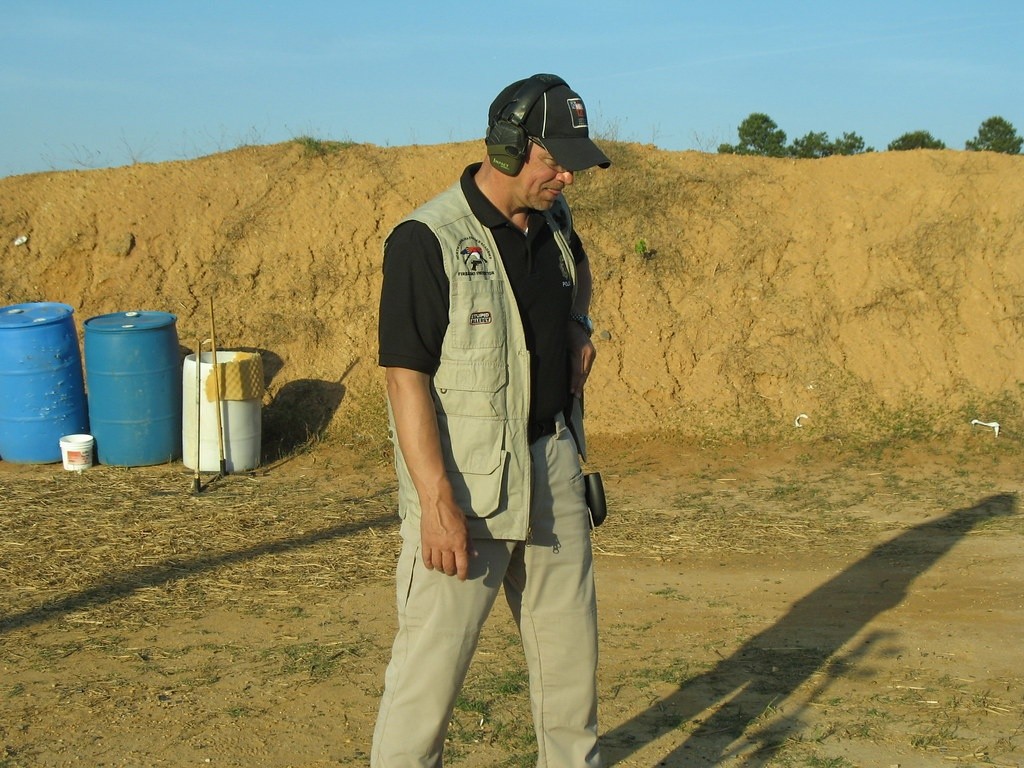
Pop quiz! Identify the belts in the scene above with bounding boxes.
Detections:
[529,415,570,444]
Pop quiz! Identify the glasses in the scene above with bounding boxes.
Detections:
[533,140,569,173]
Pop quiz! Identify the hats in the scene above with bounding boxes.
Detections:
[489,78,611,171]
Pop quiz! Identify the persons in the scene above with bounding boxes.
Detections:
[371,73,613,767]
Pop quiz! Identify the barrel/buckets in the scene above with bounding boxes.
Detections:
[182,351,262,471]
[59,434,93,471]
[0,302,87,465]
[83,311,182,466]
